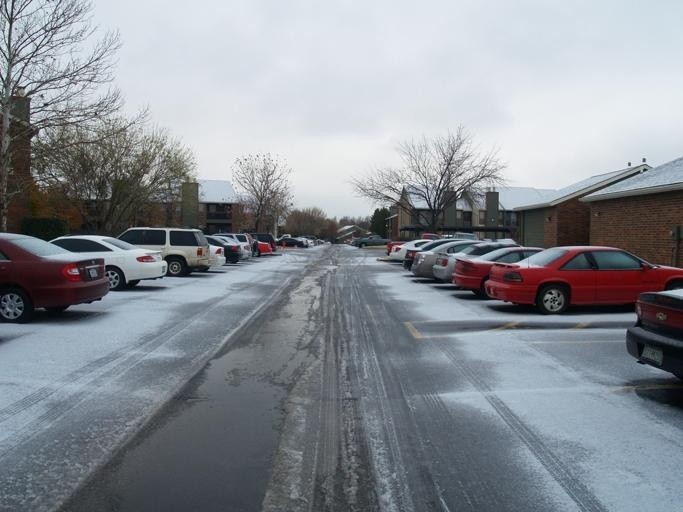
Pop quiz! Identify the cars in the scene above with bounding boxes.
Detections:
[277,238,303,248]
[625,286,683,384]
[46,234,168,291]
[214,236,251,260]
[389,239,434,262]
[450,247,546,299]
[0,233,110,323]
[403,240,452,271]
[410,240,484,281]
[205,236,241,263]
[192,242,226,272]
[432,242,521,283]
[483,245,683,314]
[253,238,273,257]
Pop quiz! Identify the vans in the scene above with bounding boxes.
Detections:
[250,234,276,251]
[212,234,252,250]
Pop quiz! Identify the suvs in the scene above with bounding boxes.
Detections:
[115,226,210,277]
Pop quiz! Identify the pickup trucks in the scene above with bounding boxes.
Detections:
[352,234,392,250]
